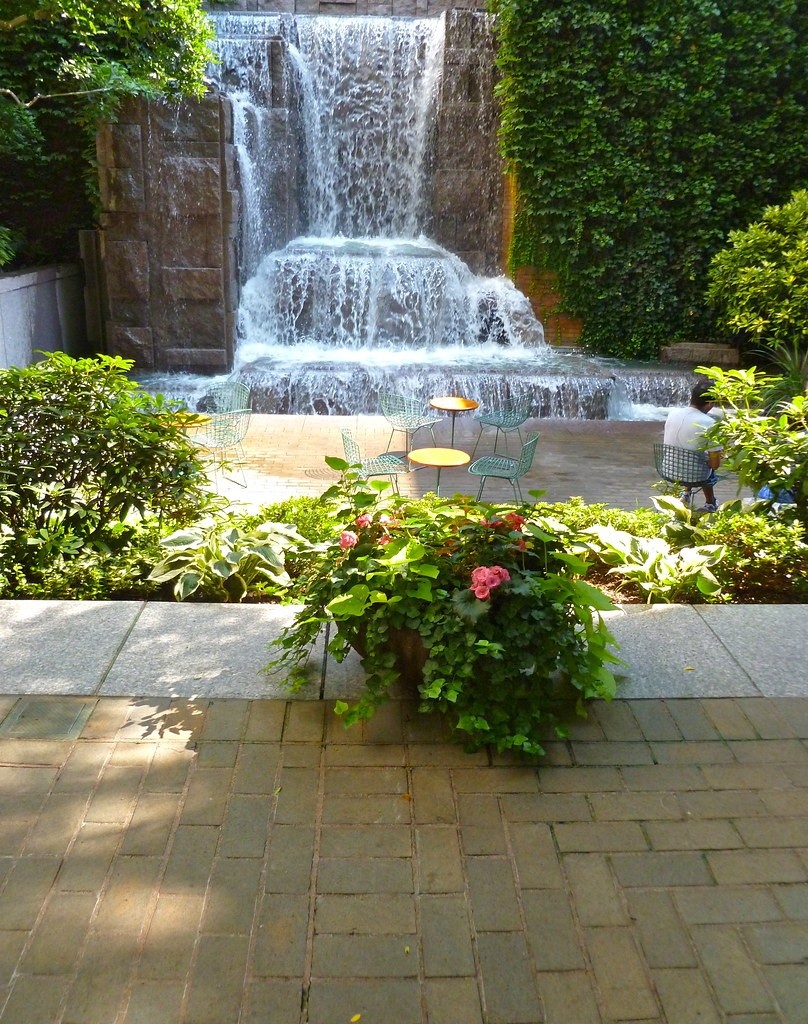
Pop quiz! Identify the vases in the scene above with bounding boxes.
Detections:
[331,610,434,675]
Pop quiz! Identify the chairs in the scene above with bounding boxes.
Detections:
[468,430,541,506]
[378,392,444,473]
[185,408,252,488]
[470,391,534,471]
[194,379,252,465]
[653,443,714,514]
[341,429,410,499]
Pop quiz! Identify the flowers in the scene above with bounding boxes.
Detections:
[256,455,629,775]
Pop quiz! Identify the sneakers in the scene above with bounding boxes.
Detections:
[788,487,799,502]
[680,491,691,507]
[703,499,720,511]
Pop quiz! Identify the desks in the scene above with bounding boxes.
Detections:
[429,396,479,449]
[146,411,214,429]
[407,447,471,498]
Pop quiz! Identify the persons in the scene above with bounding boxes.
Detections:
[662,381,720,512]
[757,412,800,503]
